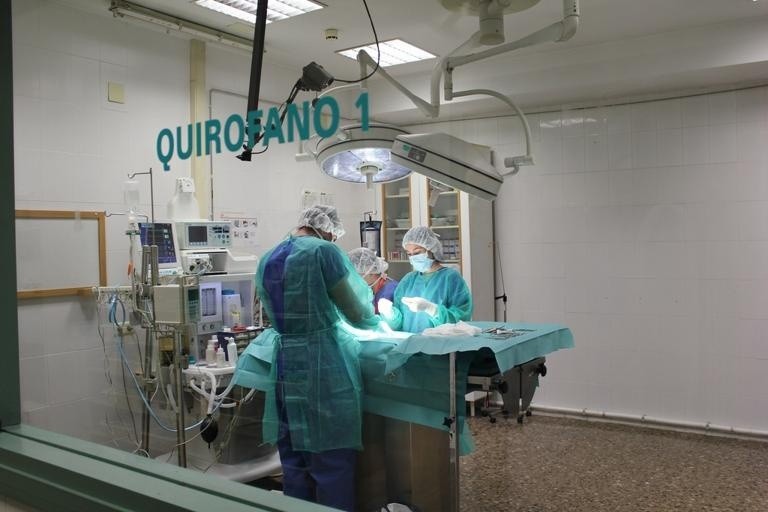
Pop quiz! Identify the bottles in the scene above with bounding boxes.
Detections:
[205,334,238,369]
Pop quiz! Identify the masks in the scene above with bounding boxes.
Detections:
[407,251,436,274]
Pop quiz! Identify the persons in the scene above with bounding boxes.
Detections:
[254,204,386,511]
[342,246,397,315]
[378,226,473,334]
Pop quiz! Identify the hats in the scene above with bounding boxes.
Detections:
[401,224,443,261]
[296,204,346,240]
[347,247,390,275]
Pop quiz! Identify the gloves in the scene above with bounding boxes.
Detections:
[401,297,438,317]
[376,297,394,319]
